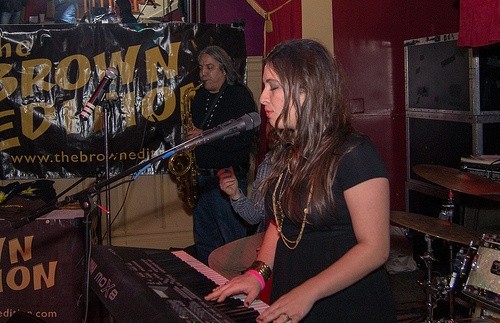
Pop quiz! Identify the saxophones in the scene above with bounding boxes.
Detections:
[166,80,208,213]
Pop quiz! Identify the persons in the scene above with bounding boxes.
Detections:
[204,38,396,323]
[185,45,260,268]
[208,119,277,279]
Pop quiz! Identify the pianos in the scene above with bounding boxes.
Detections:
[90,246,295,323]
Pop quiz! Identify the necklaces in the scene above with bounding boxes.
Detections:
[205,92,224,131]
[272,172,314,250]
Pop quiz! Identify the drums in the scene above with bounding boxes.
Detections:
[461,233,500,307]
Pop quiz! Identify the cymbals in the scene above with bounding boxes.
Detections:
[410,163,500,198]
[388,209,479,246]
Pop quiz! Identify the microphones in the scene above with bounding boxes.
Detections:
[80,67,118,122]
[175,113,261,152]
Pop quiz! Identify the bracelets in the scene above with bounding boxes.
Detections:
[245,261,272,290]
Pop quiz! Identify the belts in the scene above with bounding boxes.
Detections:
[200,170,217,176]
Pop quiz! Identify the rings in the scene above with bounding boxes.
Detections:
[225,281,228,284]
[282,312,290,320]
